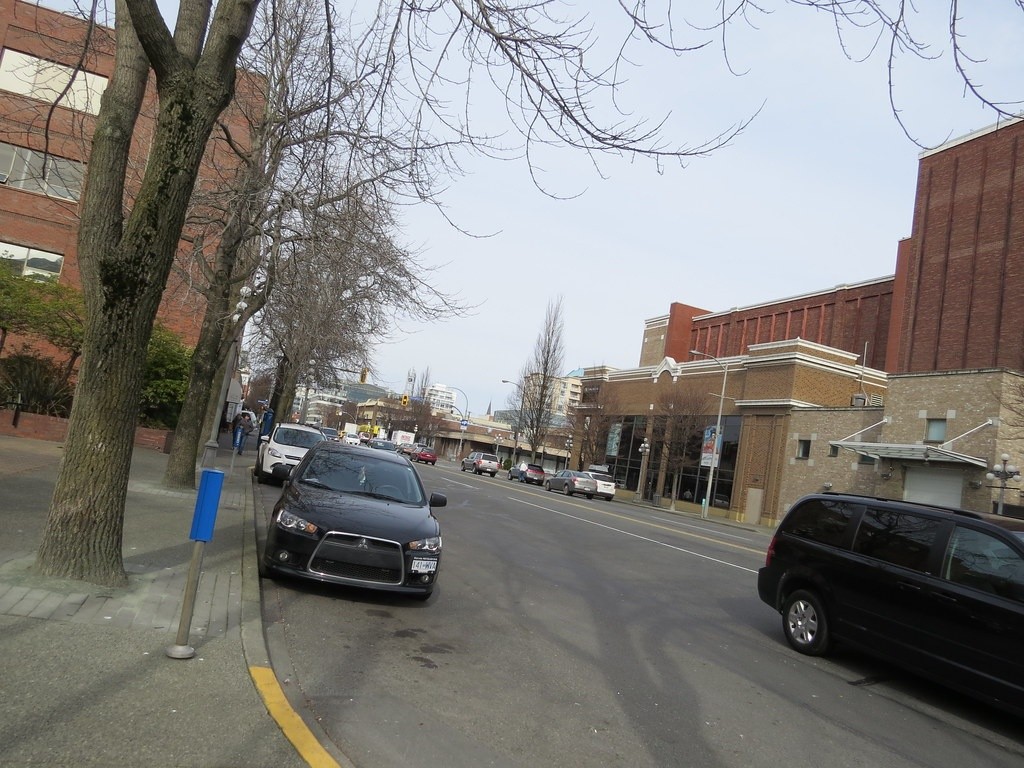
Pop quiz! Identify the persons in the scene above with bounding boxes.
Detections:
[519,461,527,482]
[499,456,505,469]
[232,413,254,456]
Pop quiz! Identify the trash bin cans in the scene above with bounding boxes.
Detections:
[653,495,660,507]
[503,458,512,470]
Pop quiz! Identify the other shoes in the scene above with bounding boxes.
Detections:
[238,451,241,455]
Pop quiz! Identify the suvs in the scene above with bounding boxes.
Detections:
[507,461,545,486]
[757,491,1024,720]
[460,451,501,477]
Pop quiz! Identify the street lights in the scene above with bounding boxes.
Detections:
[502,379,526,466]
[199,285,252,466]
[690,349,729,514]
[638,437,650,501]
[985,453,1022,515]
[297,347,320,426]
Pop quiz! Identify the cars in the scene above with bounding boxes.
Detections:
[253,421,330,485]
[582,472,615,501]
[413,446,438,465]
[710,493,730,508]
[302,422,428,456]
[545,469,598,499]
[615,477,628,490]
[258,440,448,602]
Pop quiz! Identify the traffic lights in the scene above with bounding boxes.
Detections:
[336,407,341,416]
[401,394,408,406]
